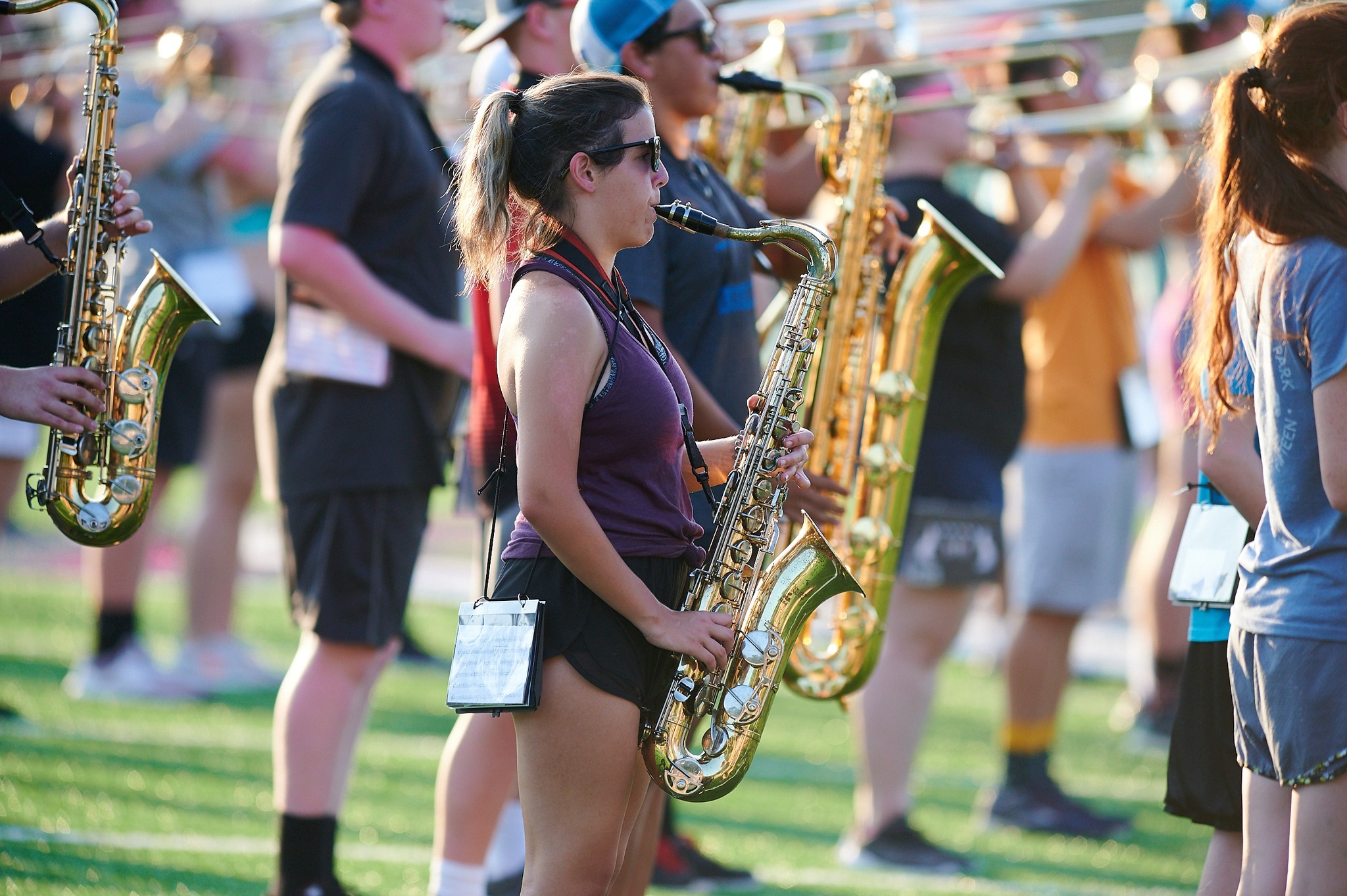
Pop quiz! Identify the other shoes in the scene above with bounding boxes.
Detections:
[649,829,753,888]
[167,628,272,697]
[841,813,974,876]
[984,779,1127,841]
[63,637,192,702]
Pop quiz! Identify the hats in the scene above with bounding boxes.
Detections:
[567,0,677,75]
[456,0,533,53]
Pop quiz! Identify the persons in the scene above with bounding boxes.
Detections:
[1110,0,1272,754]
[460,70,816,894]
[1,154,153,433]
[426,1,588,892]
[845,61,1129,868]
[984,21,1217,844]
[252,0,473,896]
[0,0,281,697]
[569,0,916,888]
[1162,1,1345,895]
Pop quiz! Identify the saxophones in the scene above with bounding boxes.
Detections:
[643,199,866,804]
[0,1,220,547]
[716,67,1006,699]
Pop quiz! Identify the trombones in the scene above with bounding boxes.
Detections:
[703,0,1271,135]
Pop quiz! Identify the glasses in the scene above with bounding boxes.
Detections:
[558,136,661,181]
[656,18,717,55]
